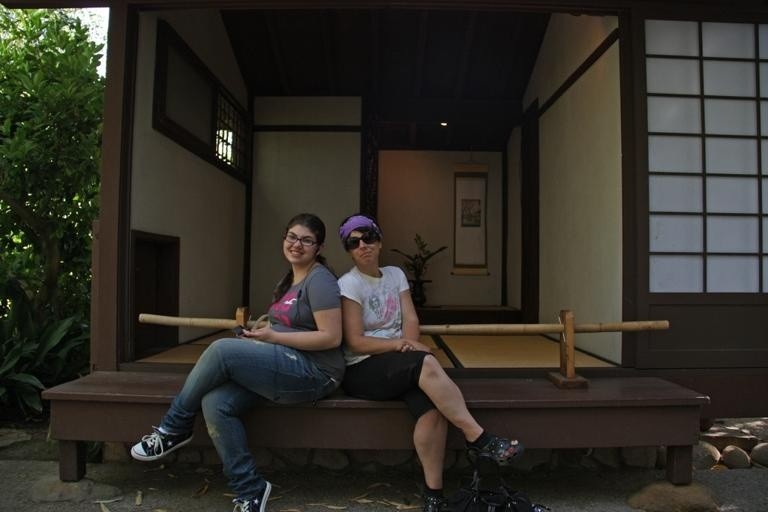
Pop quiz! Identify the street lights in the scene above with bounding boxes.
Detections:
[448,489,531,512]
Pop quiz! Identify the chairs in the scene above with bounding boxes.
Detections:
[423,496,445,512]
[232,480,272,512]
[131,425,196,462]
[462,436,523,468]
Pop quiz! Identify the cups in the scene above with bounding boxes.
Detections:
[283,232,320,246]
[344,231,381,249]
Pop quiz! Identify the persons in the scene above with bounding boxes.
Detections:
[132,213,345,510]
[336,215,521,512]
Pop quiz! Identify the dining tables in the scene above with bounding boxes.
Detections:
[390,233,448,306]
